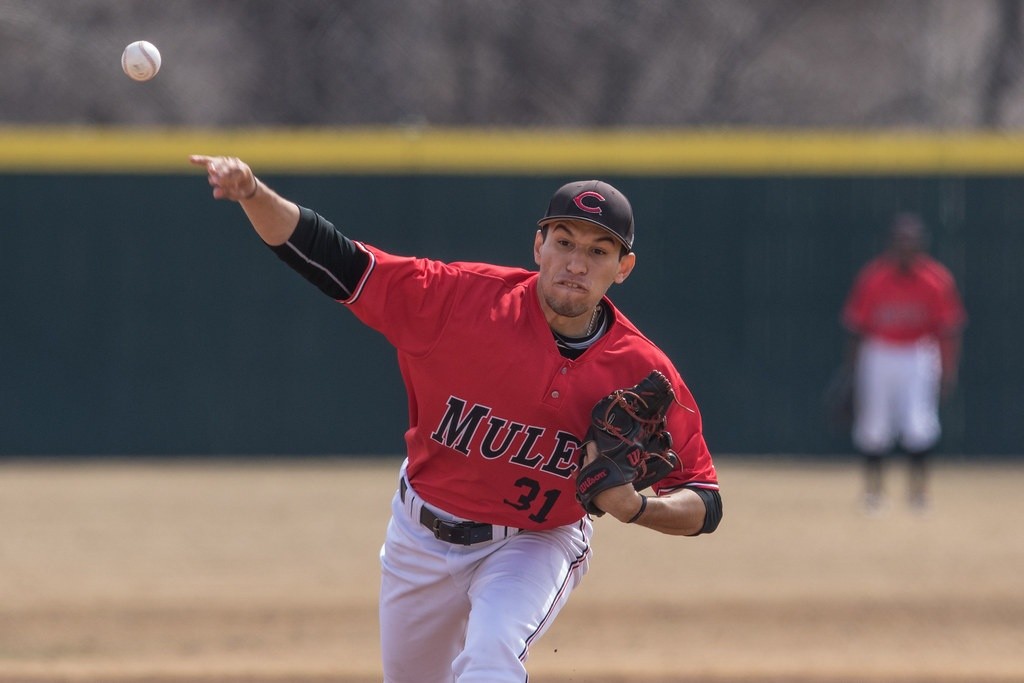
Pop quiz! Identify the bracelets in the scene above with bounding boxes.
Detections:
[248,177,257,198]
[627,494,647,523]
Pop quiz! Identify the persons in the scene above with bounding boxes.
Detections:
[190,156,723,682]
[841,212,961,519]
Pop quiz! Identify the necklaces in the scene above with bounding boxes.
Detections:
[587,310,596,335]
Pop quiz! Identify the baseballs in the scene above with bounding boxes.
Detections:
[119,40,162,82]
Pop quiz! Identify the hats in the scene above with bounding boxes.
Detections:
[537,180,635,250]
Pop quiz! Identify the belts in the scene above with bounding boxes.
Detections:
[401,478,524,546]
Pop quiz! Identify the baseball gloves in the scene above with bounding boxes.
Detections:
[574,369,695,522]
